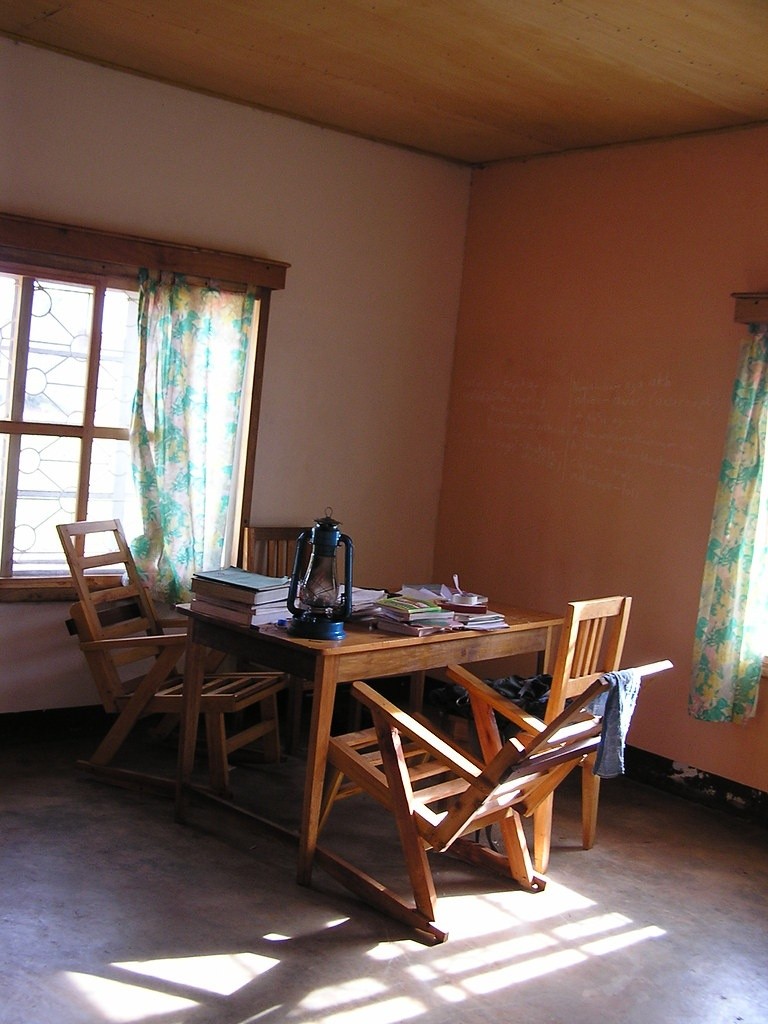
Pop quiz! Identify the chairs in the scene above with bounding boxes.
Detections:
[55,519,290,801]
[237,526,364,756]
[447,595,632,849]
[313,660,673,948]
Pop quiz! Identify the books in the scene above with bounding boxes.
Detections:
[335,583,511,637]
[189,566,300,626]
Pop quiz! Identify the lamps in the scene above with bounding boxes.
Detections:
[285,505,352,641]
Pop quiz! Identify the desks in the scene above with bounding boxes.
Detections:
[174,601,565,887]
